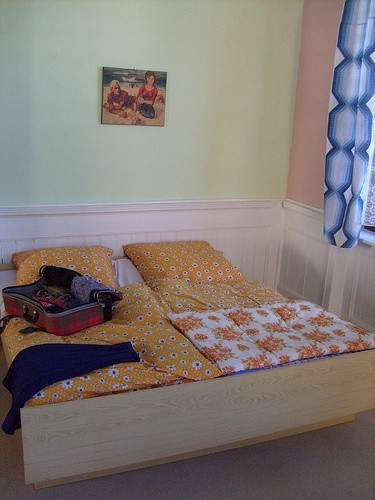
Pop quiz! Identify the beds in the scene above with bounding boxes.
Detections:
[0,249,375,489]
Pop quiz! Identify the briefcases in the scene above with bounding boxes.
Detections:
[0,265,124,336]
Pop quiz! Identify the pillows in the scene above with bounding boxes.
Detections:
[12,245,119,288]
[123,240,245,282]
